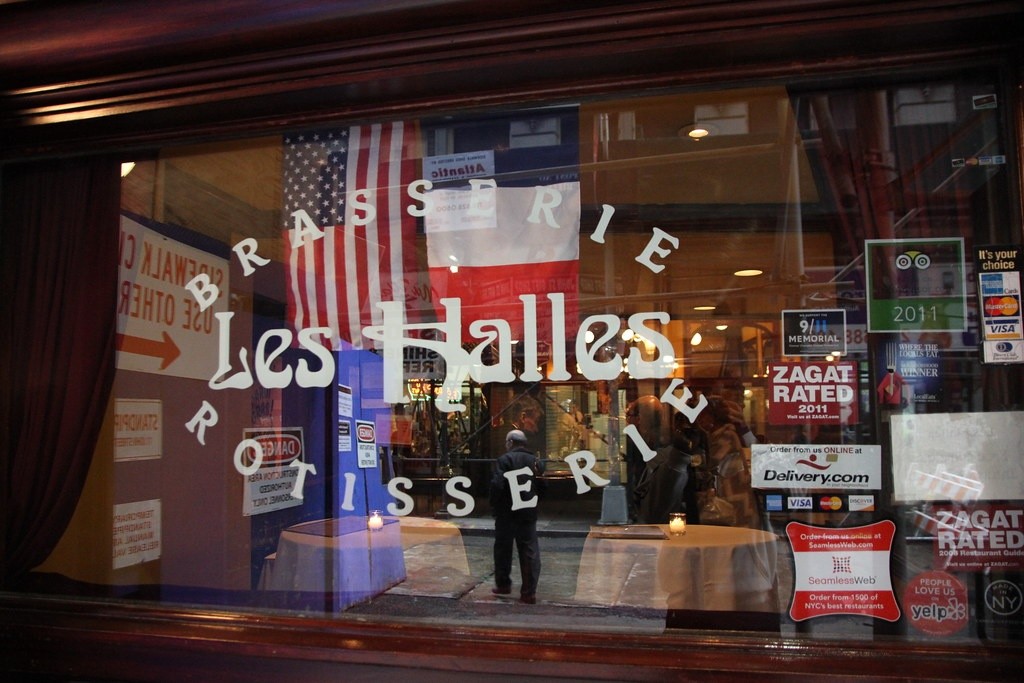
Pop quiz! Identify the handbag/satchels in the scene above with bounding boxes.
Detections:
[698,496,737,524]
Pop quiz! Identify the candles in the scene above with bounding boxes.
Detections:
[368,509,383,532]
[669,512,686,535]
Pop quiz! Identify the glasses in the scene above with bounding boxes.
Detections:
[503,440,510,444]
[624,409,642,420]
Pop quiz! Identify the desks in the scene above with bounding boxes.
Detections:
[579,522,781,611]
[275,515,471,599]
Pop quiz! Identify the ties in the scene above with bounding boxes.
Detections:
[575,412,578,423]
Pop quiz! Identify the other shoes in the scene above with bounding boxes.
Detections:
[520,592,536,603]
[492,587,512,593]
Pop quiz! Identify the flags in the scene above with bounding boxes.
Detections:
[281,119,421,350]
[421,106,581,339]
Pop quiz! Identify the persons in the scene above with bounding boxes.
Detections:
[562,399,583,447]
[492,395,544,457]
[629,395,761,529]
[488,429,543,603]
